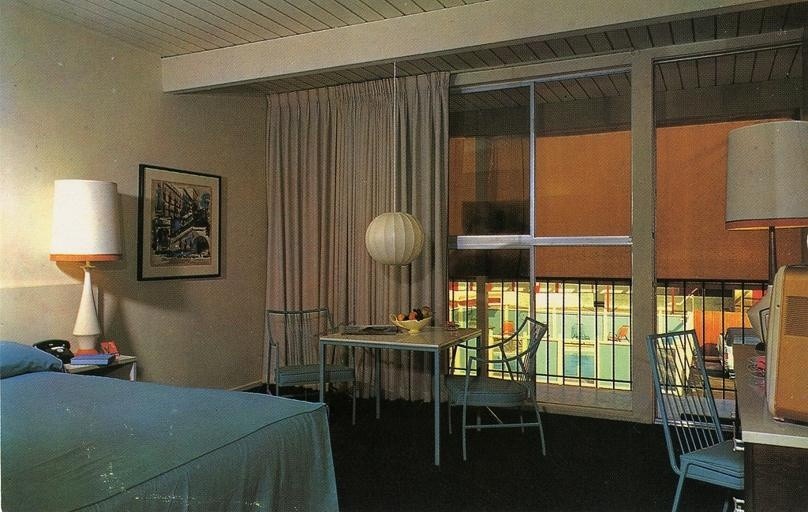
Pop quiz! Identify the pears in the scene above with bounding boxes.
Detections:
[421,308,430,318]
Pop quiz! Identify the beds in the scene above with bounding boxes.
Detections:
[0,337,338,511]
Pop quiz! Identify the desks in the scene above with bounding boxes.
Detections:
[317,324,483,466]
[724,342,808,511]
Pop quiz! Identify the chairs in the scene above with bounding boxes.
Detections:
[638,330,743,511]
[439,315,548,466]
[262,307,358,428]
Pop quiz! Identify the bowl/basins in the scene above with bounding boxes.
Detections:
[389,312,433,334]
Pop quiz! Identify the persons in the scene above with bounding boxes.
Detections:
[494,320,523,353]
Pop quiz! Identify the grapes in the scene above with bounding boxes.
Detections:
[413,309,423,321]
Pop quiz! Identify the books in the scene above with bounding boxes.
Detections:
[339,324,399,336]
[70,354,115,366]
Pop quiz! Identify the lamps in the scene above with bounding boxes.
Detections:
[44,175,126,352]
[720,121,807,340]
[364,62,427,266]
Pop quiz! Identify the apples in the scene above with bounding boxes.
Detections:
[408,312,417,320]
[398,314,405,321]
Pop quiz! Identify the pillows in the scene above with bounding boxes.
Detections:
[1,340,64,376]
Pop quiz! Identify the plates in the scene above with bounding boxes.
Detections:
[747,355,766,379]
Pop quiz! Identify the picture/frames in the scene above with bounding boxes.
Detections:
[135,164,223,282]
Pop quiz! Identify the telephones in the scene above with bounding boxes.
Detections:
[33,340,75,364]
